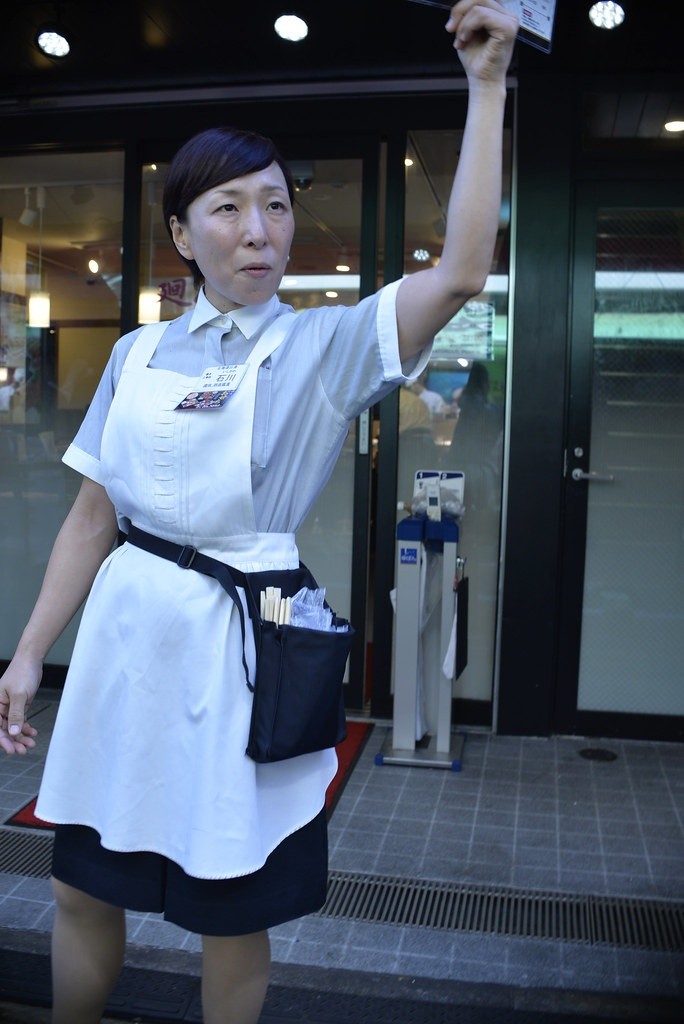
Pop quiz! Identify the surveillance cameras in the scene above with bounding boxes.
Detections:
[294,177,312,193]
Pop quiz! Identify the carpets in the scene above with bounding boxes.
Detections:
[3,719,377,833]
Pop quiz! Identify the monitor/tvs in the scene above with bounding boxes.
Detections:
[422,360,490,415]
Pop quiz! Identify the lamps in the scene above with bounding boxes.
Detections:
[34,7,70,58]
[88,249,104,273]
[28,186,51,328]
[404,147,414,166]
[19,188,36,227]
[336,246,351,271]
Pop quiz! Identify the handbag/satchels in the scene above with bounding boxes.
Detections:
[116,525,356,763]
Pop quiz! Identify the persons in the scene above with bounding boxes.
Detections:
[399,379,445,434]
[0,2,521,1022]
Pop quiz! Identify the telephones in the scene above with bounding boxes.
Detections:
[426,478,442,523]
